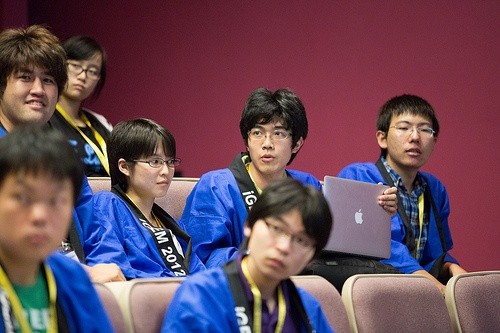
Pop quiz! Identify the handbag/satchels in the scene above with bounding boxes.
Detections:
[307,255,402,295]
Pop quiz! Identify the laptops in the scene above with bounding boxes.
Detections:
[320,176,391,259]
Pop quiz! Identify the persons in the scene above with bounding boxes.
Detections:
[336,95,469,300]
[95,119,207,282]
[160,179,336,333]
[0,26,114,333]
[180,87,397,276]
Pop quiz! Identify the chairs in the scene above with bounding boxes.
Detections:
[90,175,199,222]
[92,271,500,333]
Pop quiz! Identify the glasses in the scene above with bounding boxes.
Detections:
[129,157,183,169]
[263,216,316,255]
[247,130,291,141]
[390,125,436,138]
[67,63,101,81]
